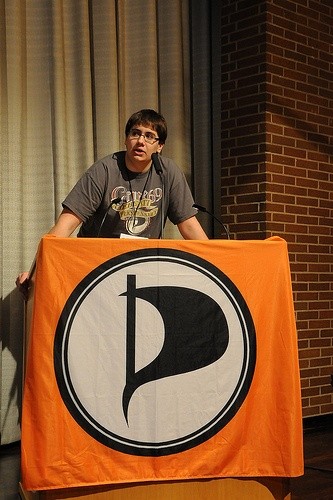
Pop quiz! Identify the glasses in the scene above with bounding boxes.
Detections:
[127,130,161,143]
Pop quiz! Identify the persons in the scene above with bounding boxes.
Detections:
[14,109,212,294]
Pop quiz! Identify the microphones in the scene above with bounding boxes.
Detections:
[192,203,231,240]
[151,153,165,239]
[95,197,121,238]
